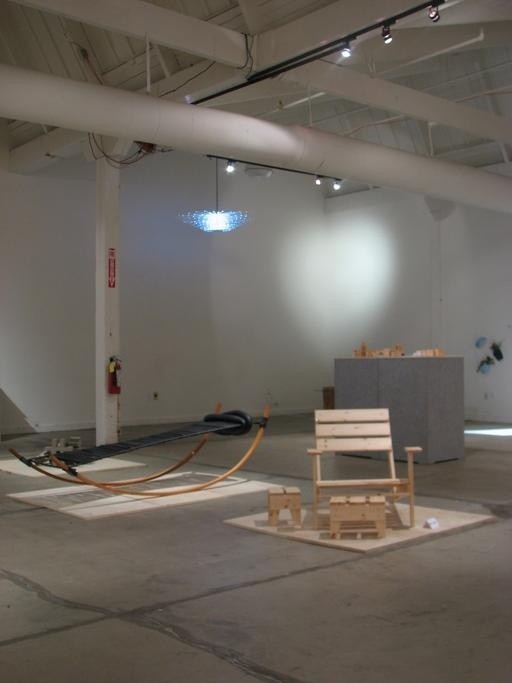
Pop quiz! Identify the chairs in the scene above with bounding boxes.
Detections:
[306,408,424,528]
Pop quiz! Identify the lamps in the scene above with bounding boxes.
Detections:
[314,174,342,191]
[340,0,447,58]
[179,153,250,232]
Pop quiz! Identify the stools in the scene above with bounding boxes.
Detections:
[267,487,301,529]
[329,494,387,541]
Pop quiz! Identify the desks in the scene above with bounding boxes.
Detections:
[335,357,465,464]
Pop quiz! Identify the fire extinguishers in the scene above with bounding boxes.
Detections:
[107,357,122,395]
